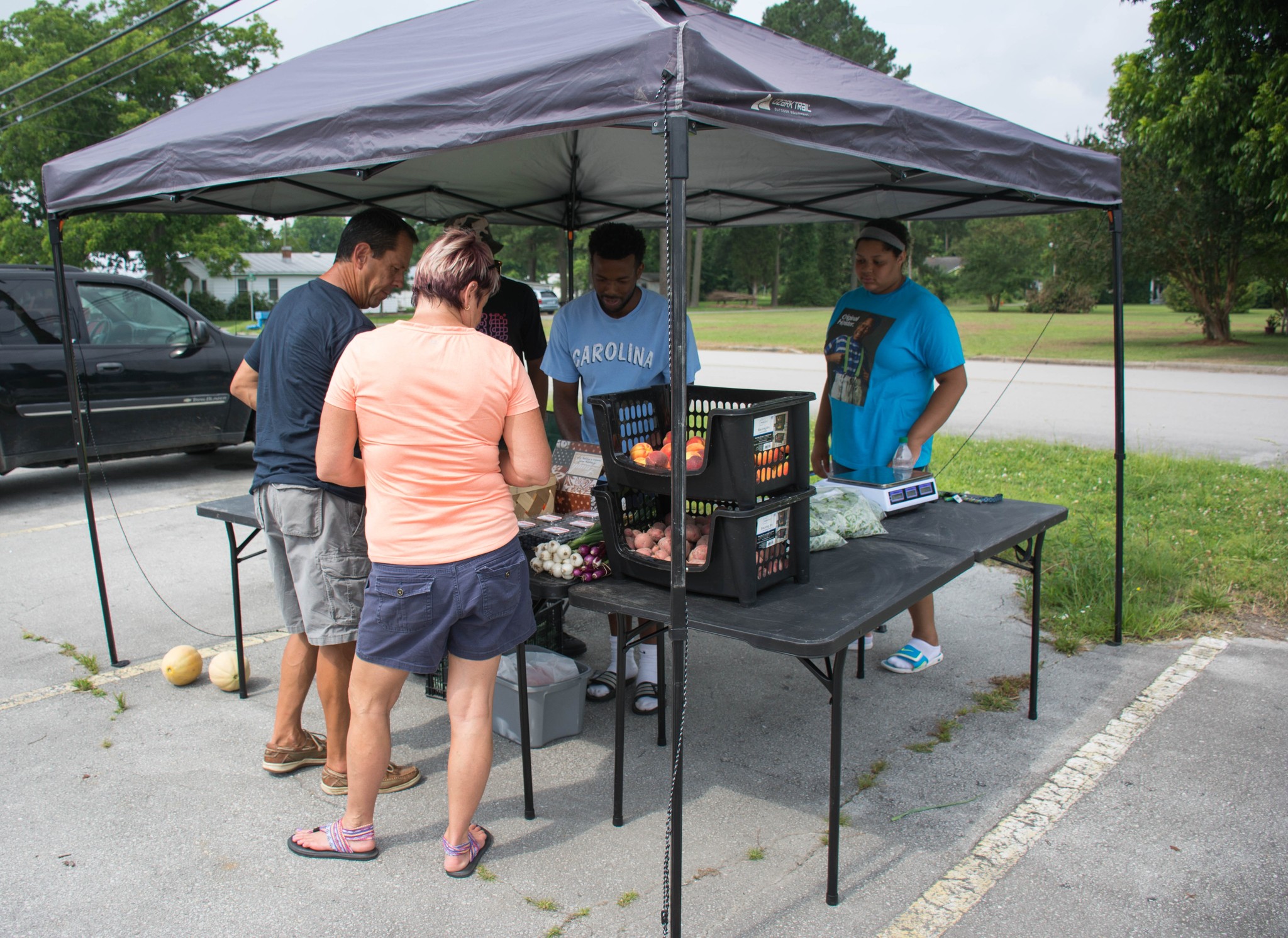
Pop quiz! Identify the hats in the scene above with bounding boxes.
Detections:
[443,213,504,255]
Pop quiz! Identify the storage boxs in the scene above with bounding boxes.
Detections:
[509,473,556,521]
[490,645,594,746]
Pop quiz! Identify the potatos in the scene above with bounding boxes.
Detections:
[624,511,787,579]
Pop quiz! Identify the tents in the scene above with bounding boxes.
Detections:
[42,0,1125,938]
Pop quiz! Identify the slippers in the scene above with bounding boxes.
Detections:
[587,671,636,700]
[880,644,944,674]
[632,681,658,716]
[847,644,872,650]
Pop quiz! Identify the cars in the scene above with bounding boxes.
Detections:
[533,288,560,315]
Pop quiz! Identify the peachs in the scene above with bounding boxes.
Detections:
[630,430,789,480]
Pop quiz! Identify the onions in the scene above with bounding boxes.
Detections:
[530,540,605,581]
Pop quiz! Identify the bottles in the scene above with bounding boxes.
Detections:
[892,467,914,483]
[892,437,914,471]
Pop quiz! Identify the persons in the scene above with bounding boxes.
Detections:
[541,223,701,715]
[288,229,552,879]
[812,219,966,674]
[229,206,422,795]
[441,214,586,656]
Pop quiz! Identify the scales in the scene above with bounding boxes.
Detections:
[814,465,939,515]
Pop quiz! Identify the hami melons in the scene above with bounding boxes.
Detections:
[208,652,251,692]
[161,644,202,685]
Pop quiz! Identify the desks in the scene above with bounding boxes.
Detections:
[567,494,1070,905]
[196,491,577,822]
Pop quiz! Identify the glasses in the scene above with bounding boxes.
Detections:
[486,259,502,275]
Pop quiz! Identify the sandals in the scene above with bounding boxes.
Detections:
[443,823,493,878]
[287,817,379,861]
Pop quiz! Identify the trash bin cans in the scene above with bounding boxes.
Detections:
[492,645,591,748]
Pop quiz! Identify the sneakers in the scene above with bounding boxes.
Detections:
[320,762,420,795]
[261,729,327,774]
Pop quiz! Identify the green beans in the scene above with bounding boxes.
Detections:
[810,491,887,551]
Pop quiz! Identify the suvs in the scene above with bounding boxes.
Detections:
[0,266,262,475]
